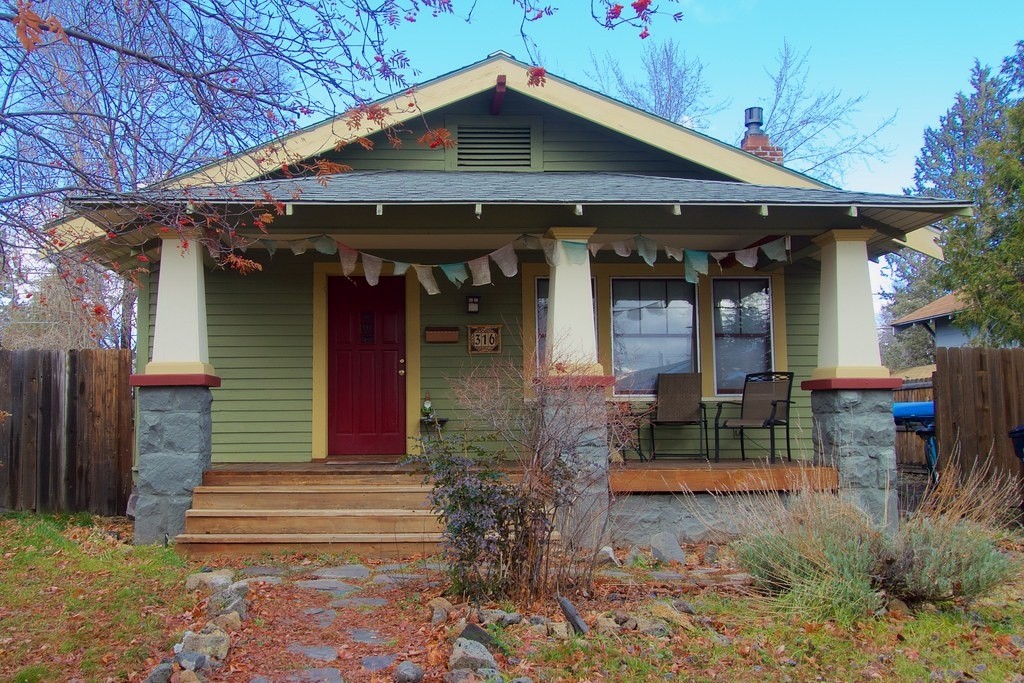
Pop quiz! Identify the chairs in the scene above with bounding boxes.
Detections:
[605,401,647,464]
[715,372,795,464]
[646,373,710,464]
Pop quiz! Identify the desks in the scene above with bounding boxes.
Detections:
[421,418,448,442]
